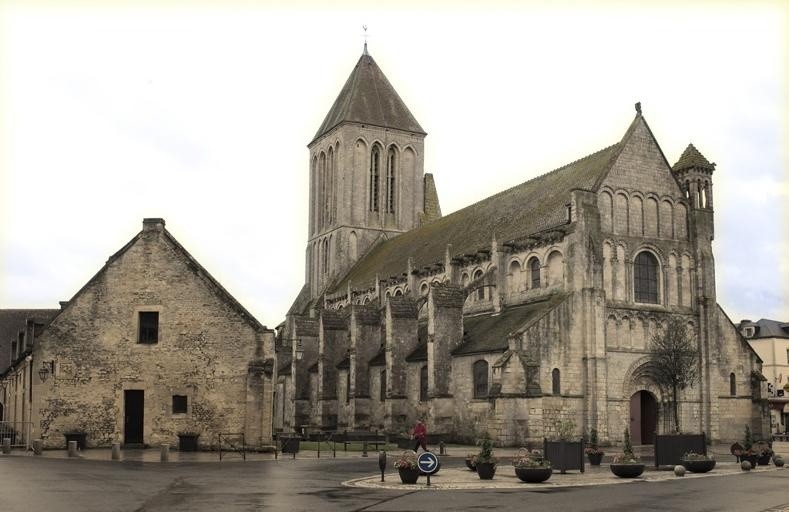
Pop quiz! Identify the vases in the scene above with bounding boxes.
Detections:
[398,470,420,484]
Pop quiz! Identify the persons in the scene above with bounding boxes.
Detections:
[411,419,430,453]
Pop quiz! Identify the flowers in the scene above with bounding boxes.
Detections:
[394,449,420,470]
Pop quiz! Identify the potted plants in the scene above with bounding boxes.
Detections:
[544,426,586,475]
[515,450,554,481]
[610,426,647,478]
[584,426,605,466]
[682,449,717,473]
[737,424,775,468]
[466,431,498,480]
